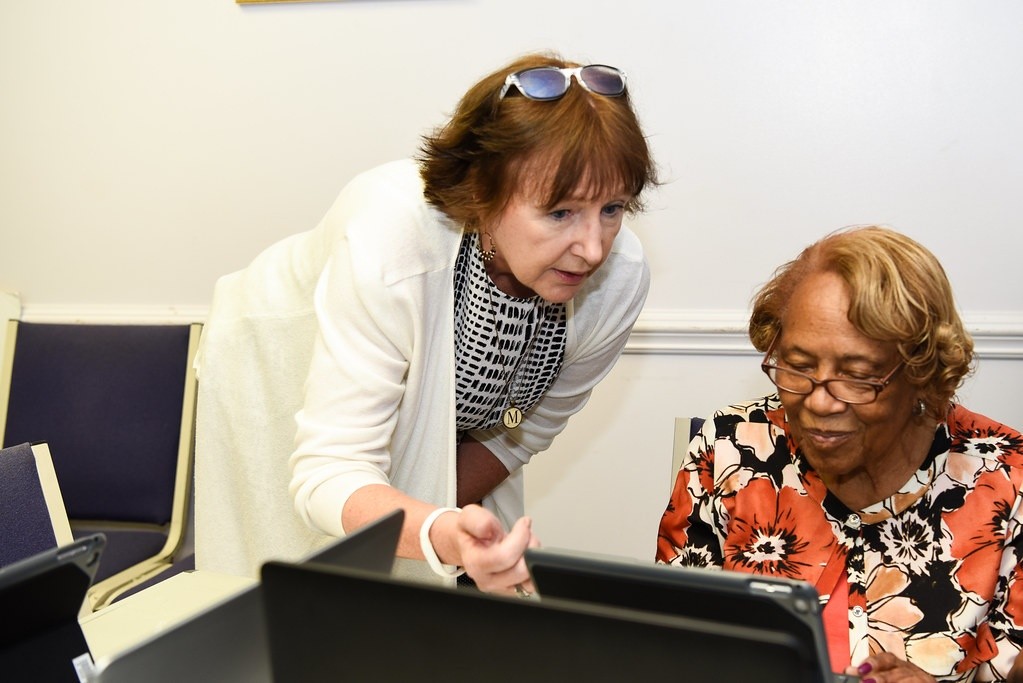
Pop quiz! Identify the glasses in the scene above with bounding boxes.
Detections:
[498,64,628,101]
[762,326,919,404]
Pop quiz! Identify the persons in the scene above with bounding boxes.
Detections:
[192,47,657,603]
[655,226,1023,683]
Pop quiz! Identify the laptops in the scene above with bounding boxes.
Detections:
[523,547,861,683]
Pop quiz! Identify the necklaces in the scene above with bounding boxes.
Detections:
[482,264,545,430]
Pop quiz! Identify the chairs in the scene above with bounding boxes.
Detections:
[0,319,204,620]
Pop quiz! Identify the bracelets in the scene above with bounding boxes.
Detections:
[419,507,466,579]
